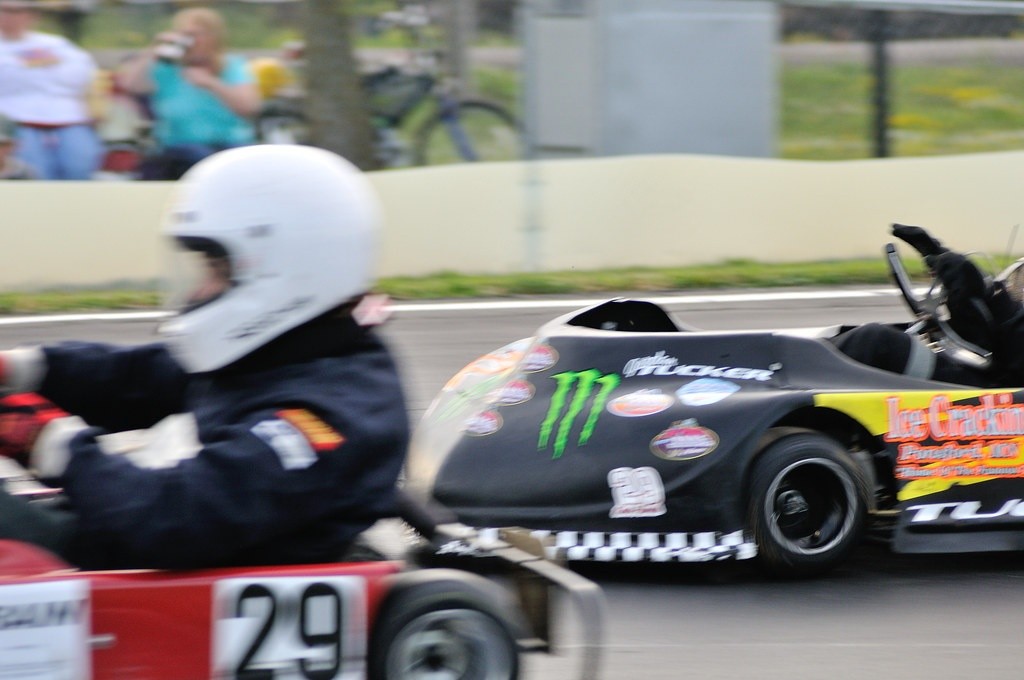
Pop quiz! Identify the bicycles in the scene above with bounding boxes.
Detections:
[252,49,536,178]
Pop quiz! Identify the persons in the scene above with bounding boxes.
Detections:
[0,144,410,564]
[0,1,263,179]
[830,223,1024,388]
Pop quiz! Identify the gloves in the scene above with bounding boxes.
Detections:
[0,393,90,480]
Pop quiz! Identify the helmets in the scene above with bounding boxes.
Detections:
[155,143,380,376]
